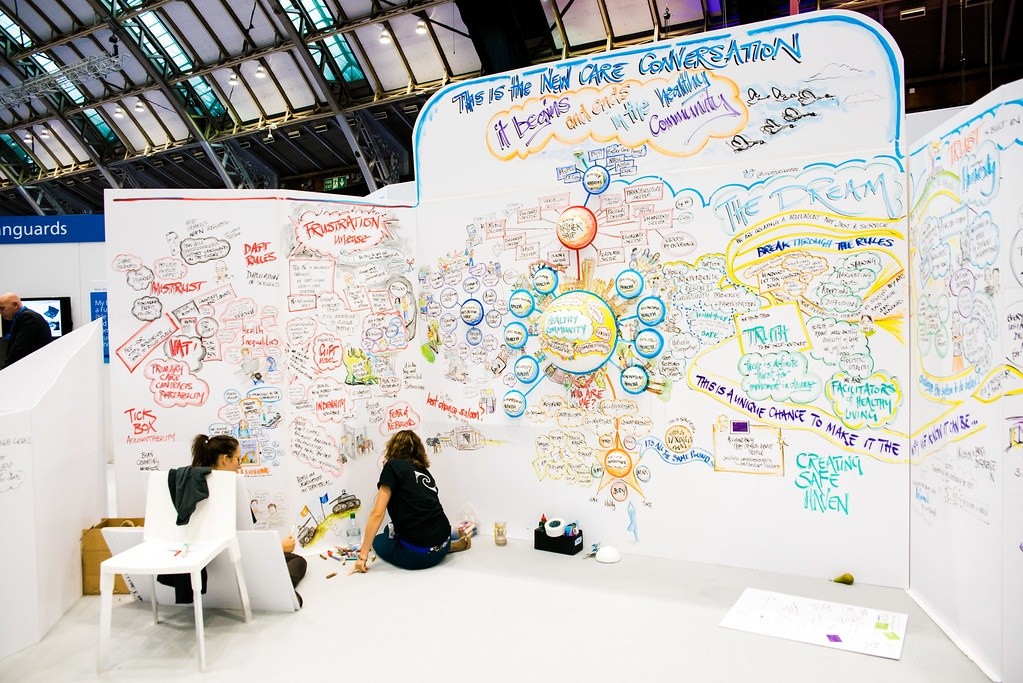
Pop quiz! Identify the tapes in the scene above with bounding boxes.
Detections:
[544,518,565,538]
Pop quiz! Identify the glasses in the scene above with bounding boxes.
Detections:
[218,455,243,465]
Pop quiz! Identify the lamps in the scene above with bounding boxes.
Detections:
[114,99,143,119]
[229,64,266,86]
[22,127,49,143]
[371,7,436,43]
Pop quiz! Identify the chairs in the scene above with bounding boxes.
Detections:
[98,468,253,674]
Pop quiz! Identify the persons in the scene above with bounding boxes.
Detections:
[347,430,472,575]
[0,292,52,372]
[192,435,306,607]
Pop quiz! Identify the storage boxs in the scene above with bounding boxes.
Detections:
[80,518,145,595]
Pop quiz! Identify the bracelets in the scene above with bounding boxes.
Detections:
[358,554,367,561]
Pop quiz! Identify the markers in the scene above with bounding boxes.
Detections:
[320,542,376,579]
[289,523,297,538]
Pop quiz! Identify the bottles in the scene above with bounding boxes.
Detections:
[347,513,361,544]
[539,513,547,531]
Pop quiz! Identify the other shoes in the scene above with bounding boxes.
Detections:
[449,534,471,552]
